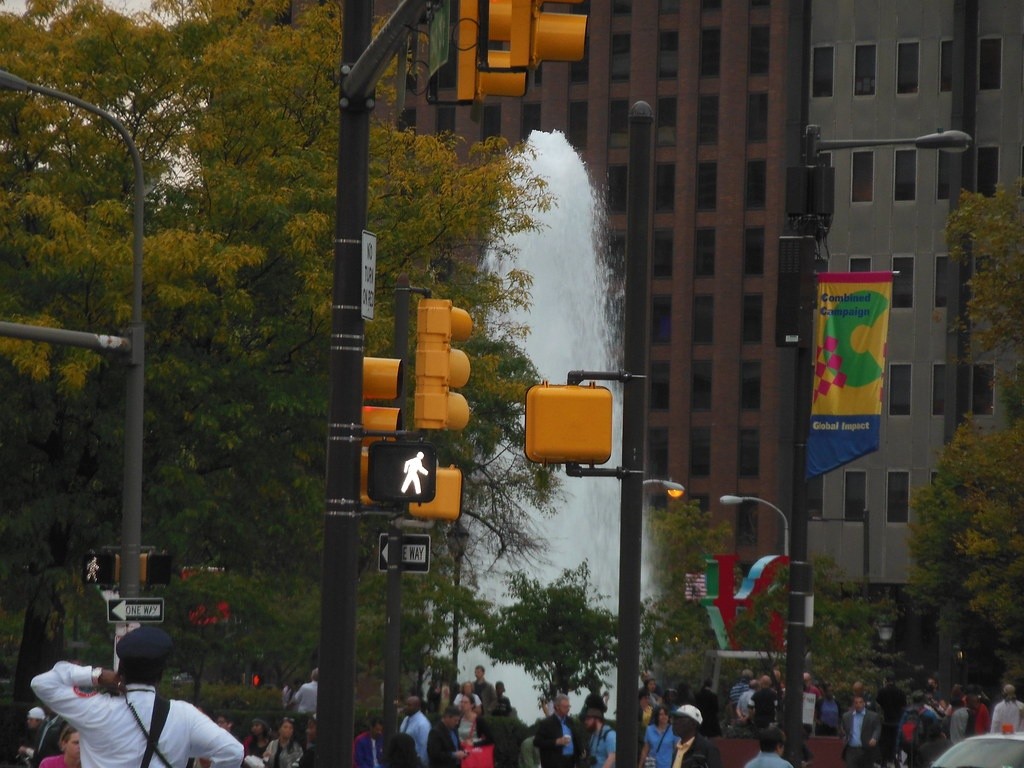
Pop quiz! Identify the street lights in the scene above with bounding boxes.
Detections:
[447,518,469,699]
[720,495,788,555]
[719,131,976,767]
[876,622,894,687]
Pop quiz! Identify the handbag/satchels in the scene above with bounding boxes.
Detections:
[644,756,656,768]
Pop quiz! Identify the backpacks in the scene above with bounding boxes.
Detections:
[900,708,926,752]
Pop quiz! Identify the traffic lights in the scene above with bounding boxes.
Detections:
[360,353,402,508]
[82,550,120,585]
[368,439,435,504]
[141,554,171,585]
[454,1,590,105]
[417,295,476,431]
[242,671,261,689]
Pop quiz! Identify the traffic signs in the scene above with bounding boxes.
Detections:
[107,598,163,622]
[378,532,431,573]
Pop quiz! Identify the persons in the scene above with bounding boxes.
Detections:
[11,662,1024,768]
[264,716,304,767]
[426,704,470,766]
[380,732,422,766]
[534,692,586,766]
[838,694,883,768]
[674,703,721,767]
[640,709,678,766]
[29,624,246,767]
[991,683,1024,735]
[580,705,617,766]
[745,725,794,768]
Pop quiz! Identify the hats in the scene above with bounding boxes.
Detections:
[117,626,175,667]
[27,706,46,719]
[670,704,703,724]
[252,717,269,731]
[586,707,602,718]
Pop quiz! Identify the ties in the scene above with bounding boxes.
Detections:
[402,718,410,734]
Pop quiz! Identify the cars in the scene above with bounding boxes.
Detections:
[927,723,1024,768]
